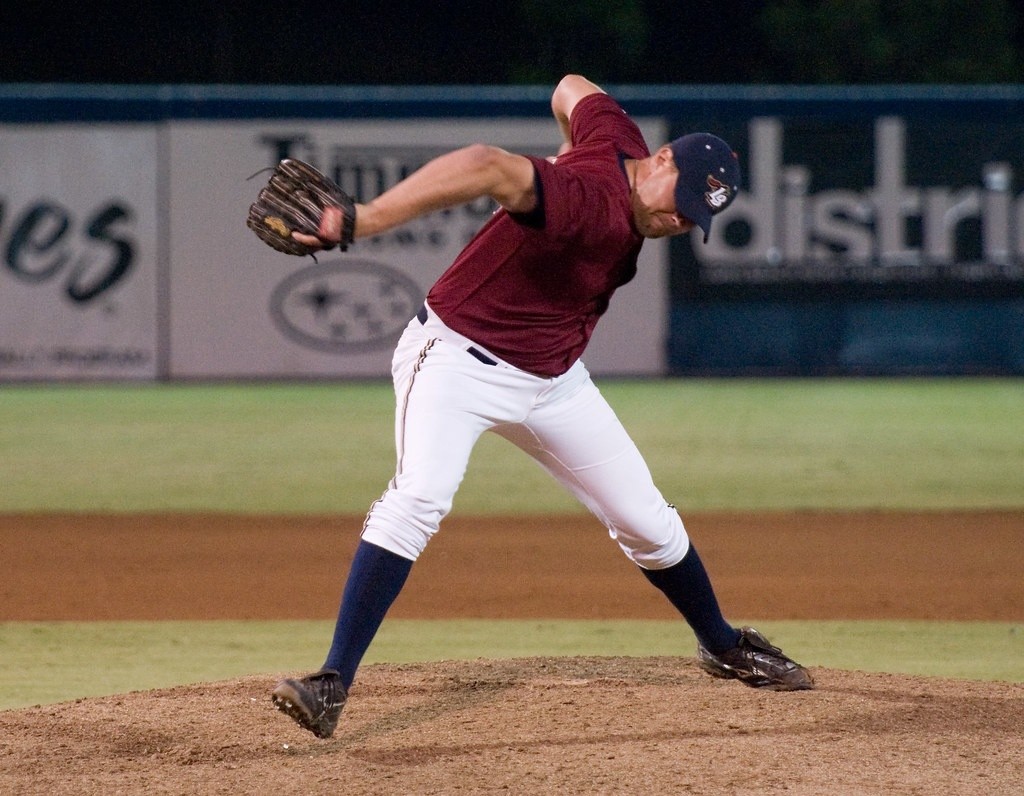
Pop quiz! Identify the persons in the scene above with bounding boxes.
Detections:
[246,74,816,738]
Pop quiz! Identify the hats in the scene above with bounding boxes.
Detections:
[672,133,742,244]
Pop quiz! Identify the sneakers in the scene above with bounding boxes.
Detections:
[271,669,349,739]
[699,626,815,691]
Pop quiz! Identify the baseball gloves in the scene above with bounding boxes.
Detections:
[246,158,356,257]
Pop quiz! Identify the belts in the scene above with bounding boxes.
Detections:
[416,304,497,366]
